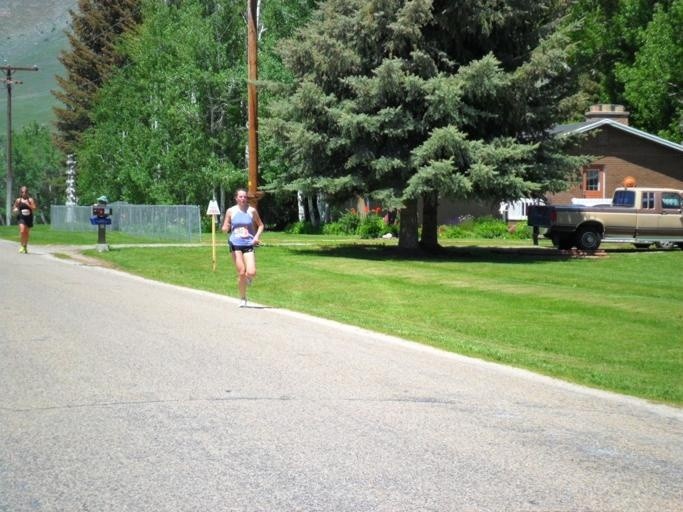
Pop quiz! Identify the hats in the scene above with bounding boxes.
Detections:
[97,195,108,203]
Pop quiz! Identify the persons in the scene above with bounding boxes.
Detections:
[12,186,36,254]
[221,188,265,307]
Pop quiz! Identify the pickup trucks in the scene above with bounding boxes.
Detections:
[527,187,683,250]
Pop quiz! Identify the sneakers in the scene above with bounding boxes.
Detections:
[245,276,252,285]
[18,245,28,254]
[239,298,247,307]
